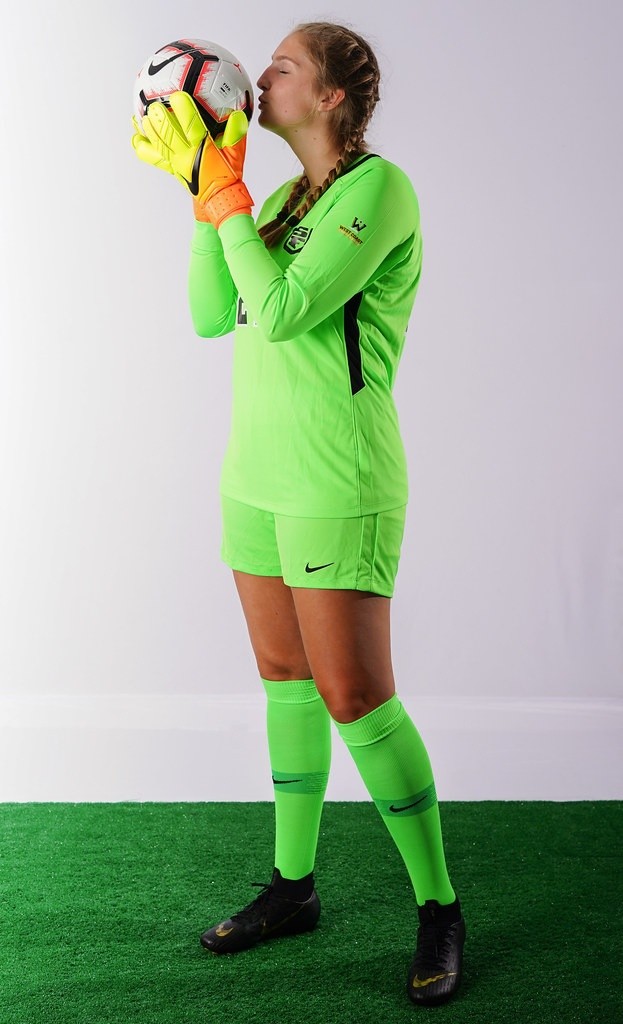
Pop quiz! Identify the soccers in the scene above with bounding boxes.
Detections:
[135,39,255,152]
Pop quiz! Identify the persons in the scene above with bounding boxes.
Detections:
[129,20,466,1007]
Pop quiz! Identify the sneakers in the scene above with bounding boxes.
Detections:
[405,918,467,1005]
[199,888,321,955]
